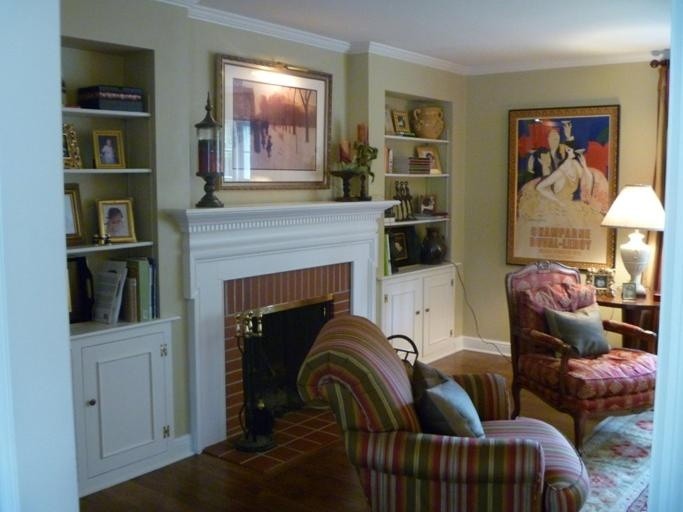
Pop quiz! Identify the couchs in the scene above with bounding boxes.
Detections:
[297,313,593,512]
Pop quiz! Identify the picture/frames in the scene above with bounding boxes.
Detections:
[392,232,408,260]
[384,108,395,133]
[214,52,333,191]
[61,121,82,171]
[91,128,126,169]
[415,144,443,175]
[95,197,136,244]
[585,267,615,298]
[504,104,619,271]
[63,182,88,248]
[391,109,409,132]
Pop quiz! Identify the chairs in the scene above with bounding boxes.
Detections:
[505,261,654,451]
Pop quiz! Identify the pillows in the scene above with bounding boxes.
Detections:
[515,282,597,354]
[427,376,490,436]
[412,360,450,434]
[544,302,613,357]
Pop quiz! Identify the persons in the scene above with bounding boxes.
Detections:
[106,207,128,237]
[426,153,437,169]
[537,145,592,207]
[101,138,116,162]
[596,276,605,288]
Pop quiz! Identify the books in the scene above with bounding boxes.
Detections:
[90,251,159,325]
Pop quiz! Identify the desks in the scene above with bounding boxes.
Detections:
[594,294,659,349]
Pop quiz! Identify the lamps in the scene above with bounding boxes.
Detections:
[598,184,665,298]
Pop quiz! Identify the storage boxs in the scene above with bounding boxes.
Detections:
[75,84,144,113]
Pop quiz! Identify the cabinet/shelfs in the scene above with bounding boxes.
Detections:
[60,34,161,337]
[381,90,453,275]
[69,315,180,499]
[377,261,456,362]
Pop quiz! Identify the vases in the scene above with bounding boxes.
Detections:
[419,226,447,266]
[412,107,446,140]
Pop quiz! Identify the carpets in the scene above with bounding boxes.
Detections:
[572,403,654,511]
[203,401,344,476]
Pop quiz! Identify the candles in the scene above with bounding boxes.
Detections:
[355,122,368,145]
[198,138,217,172]
[339,140,352,164]
